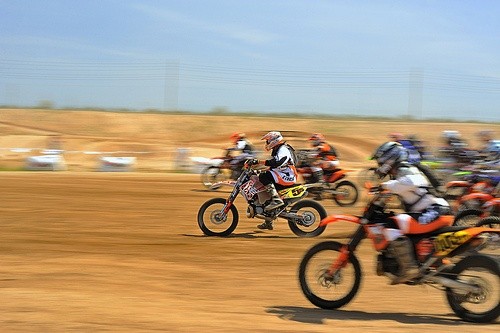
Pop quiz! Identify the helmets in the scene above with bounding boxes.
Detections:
[228,132,246,144]
[369,141,409,180]
[482,140,500,161]
[309,133,323,146]
[261,131,284,151]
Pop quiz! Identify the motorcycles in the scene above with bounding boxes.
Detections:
[199,147,249,190]
[294,158,359,207]
[359,165,395,191]
[450,191,500,252]
[427,152,500,215]
[471,197,500,258]
[296,191,500,326]
[195,158,328,239]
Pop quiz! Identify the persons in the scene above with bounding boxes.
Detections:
[364,129,500,239]
[249,130,299,230]
[304,133,341,201]
[370,141,449,286]
[225,132,252,187]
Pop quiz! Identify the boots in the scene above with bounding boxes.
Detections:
[313,170,333,200]
[265,194,284,210]
[390,240,421,286]
[257,220,273,230]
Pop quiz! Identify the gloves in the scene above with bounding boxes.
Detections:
[364,182,382,191]
[244,159,259,169]
